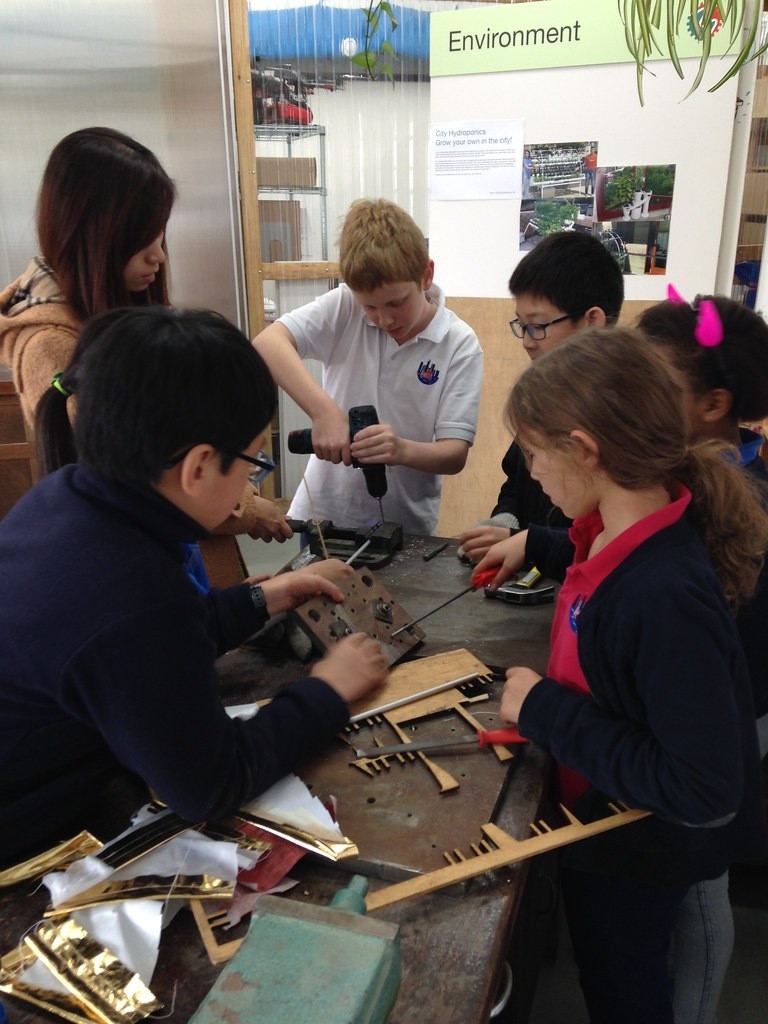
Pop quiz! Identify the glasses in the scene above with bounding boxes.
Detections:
[508,314,571,340]
[167,443,277,482]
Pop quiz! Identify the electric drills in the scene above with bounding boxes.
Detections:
[288,404,388,524]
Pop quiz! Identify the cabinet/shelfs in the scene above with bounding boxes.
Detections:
[530,149,586,199]
[253,124,328,323]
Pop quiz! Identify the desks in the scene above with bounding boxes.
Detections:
[1,529,565,1024]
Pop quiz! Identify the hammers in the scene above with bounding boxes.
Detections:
[484,565,555,604]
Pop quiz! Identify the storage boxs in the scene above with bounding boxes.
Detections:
[255,156,317,187]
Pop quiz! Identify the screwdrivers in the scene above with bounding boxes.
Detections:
[391,565,501,638]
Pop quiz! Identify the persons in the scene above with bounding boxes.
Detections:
[470,296,767,1023]
[0,127,296,590]
[249,199,482,534]
[1,307,390,869]
[582,146,597,194]
[521,149,535,199]
[460,230,625,564]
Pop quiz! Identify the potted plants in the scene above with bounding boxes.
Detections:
[616,167,654,221]
